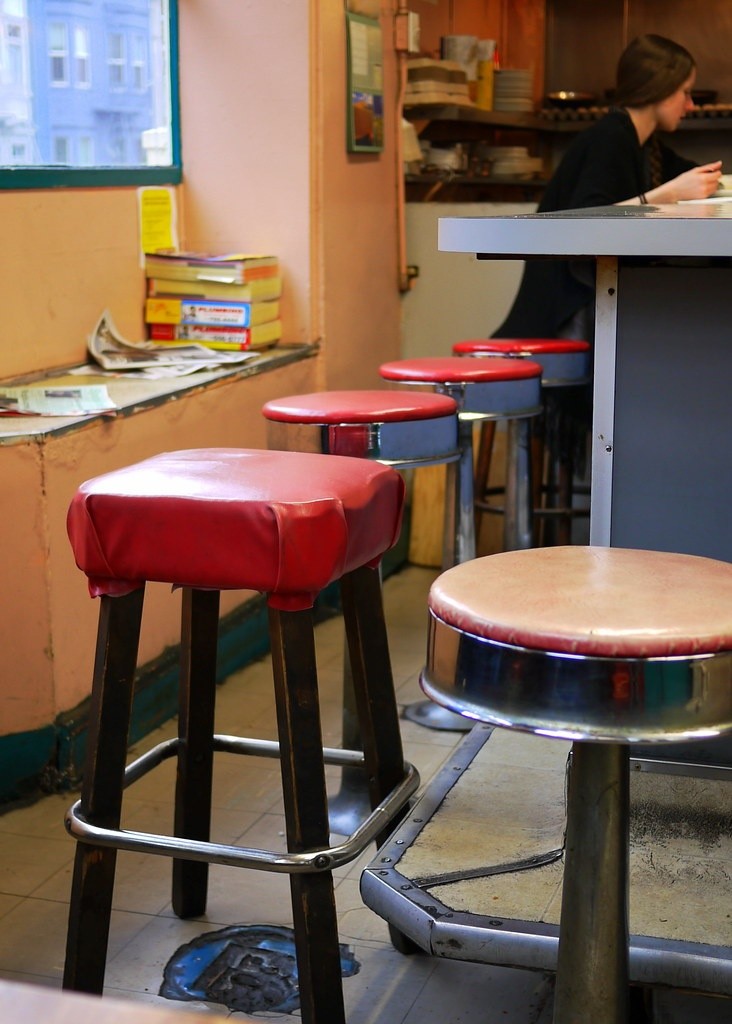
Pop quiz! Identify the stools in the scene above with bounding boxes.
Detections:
[61,444,421,1024]
[453,338,593,555]
[259,385,462,836]
[377,356,544,734]
[418,544,732,1024]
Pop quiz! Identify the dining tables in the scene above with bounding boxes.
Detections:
[436,201,732,780]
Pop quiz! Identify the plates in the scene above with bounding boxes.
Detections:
[714,174,732,197]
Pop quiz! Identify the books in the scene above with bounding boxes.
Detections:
[144,251,281,351]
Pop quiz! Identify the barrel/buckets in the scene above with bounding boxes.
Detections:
[494,69,534,111]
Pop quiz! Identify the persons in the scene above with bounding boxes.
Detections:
[490,33,722,432]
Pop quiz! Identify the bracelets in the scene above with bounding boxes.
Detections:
[639,193,647,204]
